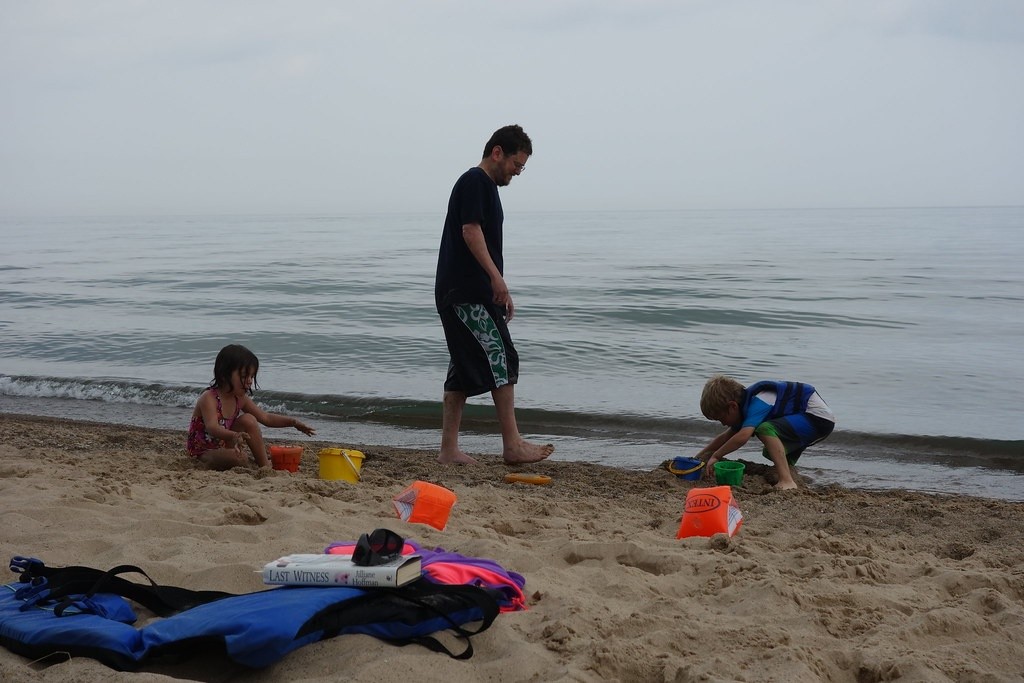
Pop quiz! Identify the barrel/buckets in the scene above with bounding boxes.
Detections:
[318,447,366,484]
[669,455,706,482]
[713,460,746,488]
[270,444,304,473]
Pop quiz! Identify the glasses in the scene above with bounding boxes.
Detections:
[504,149,526,172]
[351,528,404,566]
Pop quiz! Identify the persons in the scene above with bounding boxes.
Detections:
[187,344,316,473]
[435,125,554,466]
[693,376,836,492]
